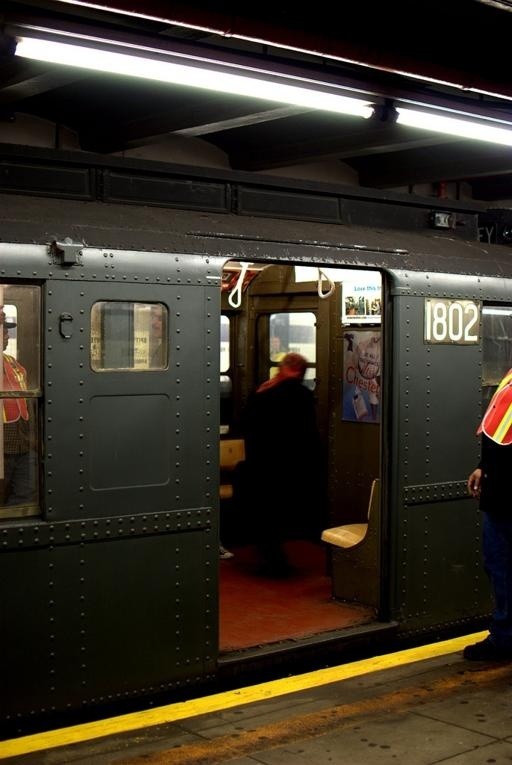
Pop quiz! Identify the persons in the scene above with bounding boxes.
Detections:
[232,351,330,578]
[464,364,511,663]
[0,305,33,504]
[371,300,381,314]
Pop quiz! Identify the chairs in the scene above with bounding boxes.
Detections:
[320,478,381,616]
[219,438,245,504]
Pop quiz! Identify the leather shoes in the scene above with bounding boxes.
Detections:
[463,639,512,661]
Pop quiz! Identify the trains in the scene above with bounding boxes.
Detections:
[1,184,510,744]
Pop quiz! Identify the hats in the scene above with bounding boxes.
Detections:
[2,311,16,328]
[279,354,307,370]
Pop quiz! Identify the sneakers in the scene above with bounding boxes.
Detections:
[218,544,235,558]
